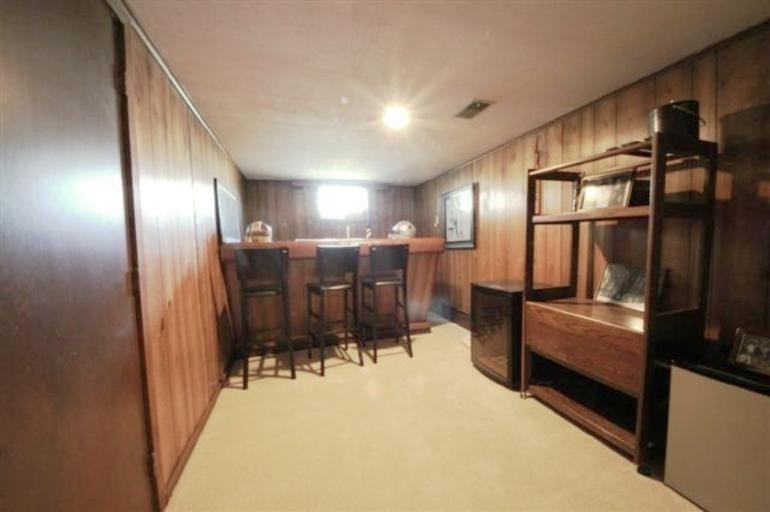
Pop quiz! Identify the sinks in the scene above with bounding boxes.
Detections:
[294,236,368,242]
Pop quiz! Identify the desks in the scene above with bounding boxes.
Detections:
[665,346,770,512]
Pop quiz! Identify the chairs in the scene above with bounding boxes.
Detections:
[235,243,413,390]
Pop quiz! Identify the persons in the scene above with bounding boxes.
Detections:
[738,341,763,367]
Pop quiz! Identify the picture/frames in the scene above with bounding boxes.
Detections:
[437,182,477,249]
[215,178,243,245]
[726,327,770,379]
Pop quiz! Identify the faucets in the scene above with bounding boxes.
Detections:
[346,226,351,239]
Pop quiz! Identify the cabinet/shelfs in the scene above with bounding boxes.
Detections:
[520,132,719,477]
[469,280,570,391]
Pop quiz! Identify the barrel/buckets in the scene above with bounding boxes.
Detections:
[651,99,705,156]
